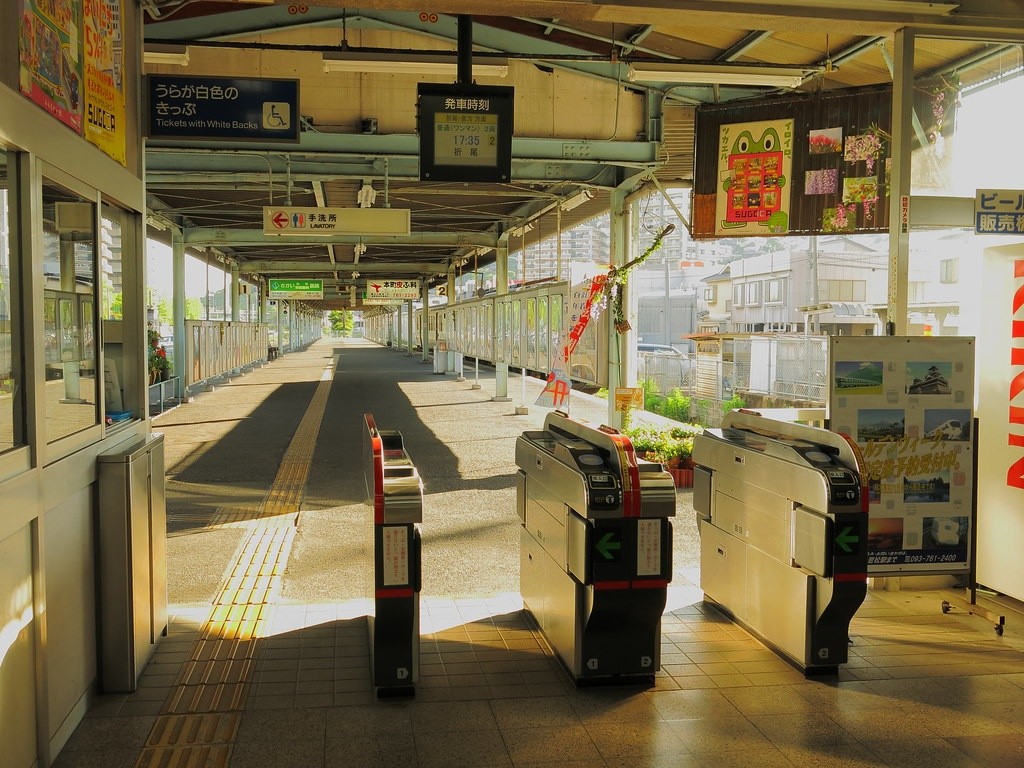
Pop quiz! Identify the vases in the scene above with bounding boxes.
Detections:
[160,367,171,381]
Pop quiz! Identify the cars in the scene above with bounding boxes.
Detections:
[635,343,697,386]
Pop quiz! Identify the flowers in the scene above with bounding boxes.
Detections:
[157,344,169,368]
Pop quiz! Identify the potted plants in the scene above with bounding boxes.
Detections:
[620,419,703,468]
[148,348,158,386]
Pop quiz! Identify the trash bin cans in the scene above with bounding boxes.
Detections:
[268,346,279,361]
[432,339,449,374]
